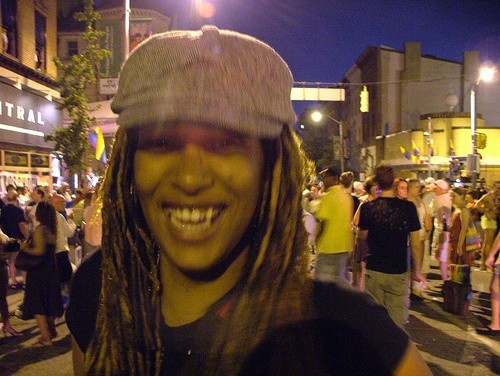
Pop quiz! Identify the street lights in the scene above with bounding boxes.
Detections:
[311,108,344,172]
[466,62,499,171]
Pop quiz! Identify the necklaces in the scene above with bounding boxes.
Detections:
[155,245,248,294]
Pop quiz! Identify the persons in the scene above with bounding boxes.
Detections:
[359,166,431,333]
[0,181,99,336]
[66,23,435,376]
[14,200,60,346]
[302,160,500,334]
[309,166,355,283]
